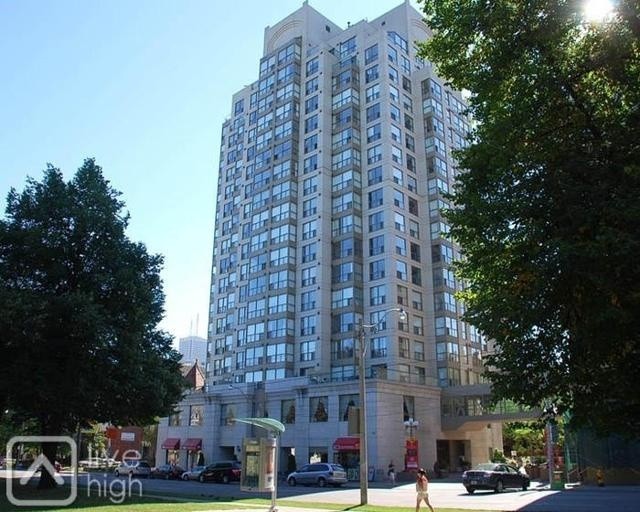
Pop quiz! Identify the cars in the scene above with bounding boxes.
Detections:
[463,462,531,493]
[198,461,241,483]
[288,462,348,487]
[181,466,206,480]
[151,465,178,480]
[79,457,121,471]
[36,460,61,472]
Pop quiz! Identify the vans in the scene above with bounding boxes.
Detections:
[114,461,151,479]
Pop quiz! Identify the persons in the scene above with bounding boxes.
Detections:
[387,459,395,486]
[415,468,434,511]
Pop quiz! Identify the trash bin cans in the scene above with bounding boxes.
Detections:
[552,471,565,490]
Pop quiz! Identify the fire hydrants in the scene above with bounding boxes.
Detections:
[595,470,606,486]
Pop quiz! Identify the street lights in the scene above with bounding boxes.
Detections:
[404,417,418,442]
[358,308,406,505]
